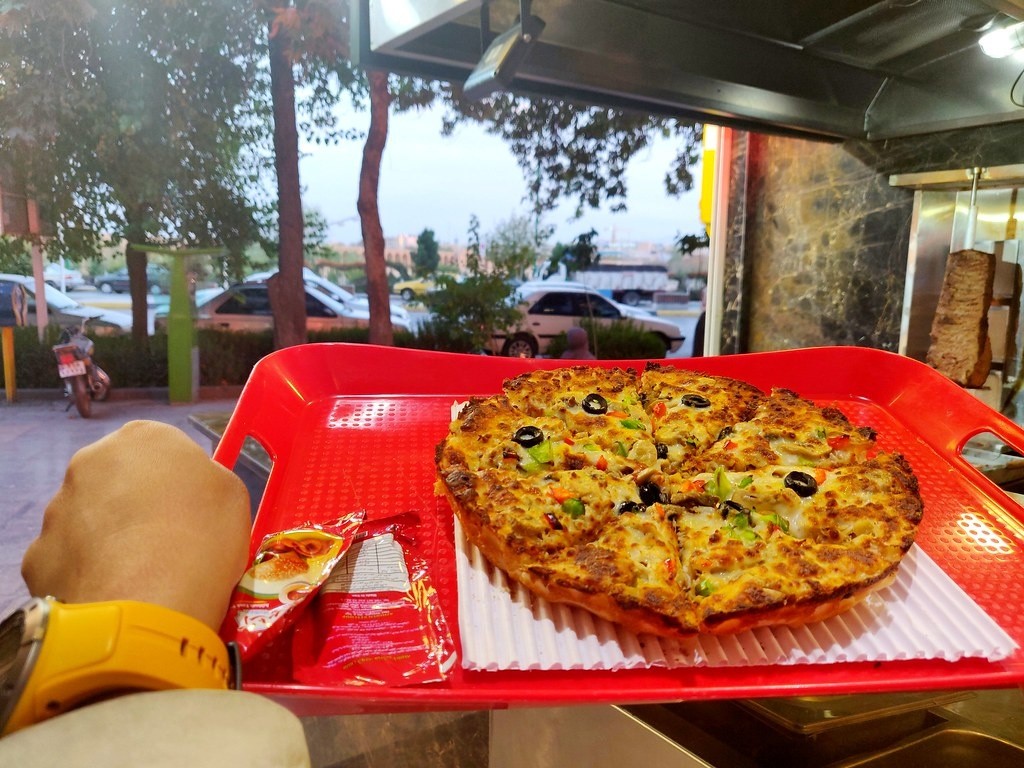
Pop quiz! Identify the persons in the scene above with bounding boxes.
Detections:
[0,418,1024,768]
[559,327,598,360]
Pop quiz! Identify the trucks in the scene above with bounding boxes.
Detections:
[530,261,667,308]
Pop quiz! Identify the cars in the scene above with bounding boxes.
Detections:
[393,271,465,300]
[95,263,201,296]
[466,282,686,360]
[149,268,411,336]
[40,261,84,290]
[0,273,134,337]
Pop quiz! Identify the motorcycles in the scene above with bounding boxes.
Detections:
[50,313,113,420]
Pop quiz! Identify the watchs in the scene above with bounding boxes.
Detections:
[0,591,245,744]
